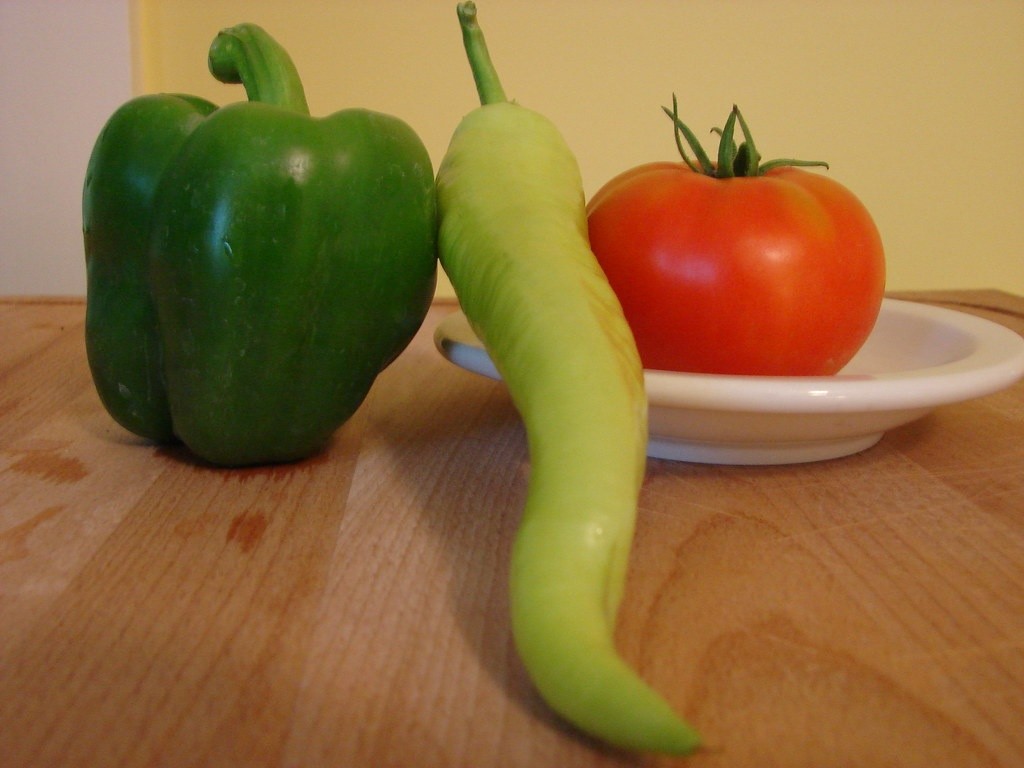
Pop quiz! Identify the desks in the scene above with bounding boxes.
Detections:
[1,287,1024,768]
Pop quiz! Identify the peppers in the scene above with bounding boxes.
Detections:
[434,0,706,755]
[82,21,439,467]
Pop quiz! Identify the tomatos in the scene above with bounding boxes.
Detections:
[584,92,887,378]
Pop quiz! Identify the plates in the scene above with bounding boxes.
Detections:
[435,294,1023,466]
